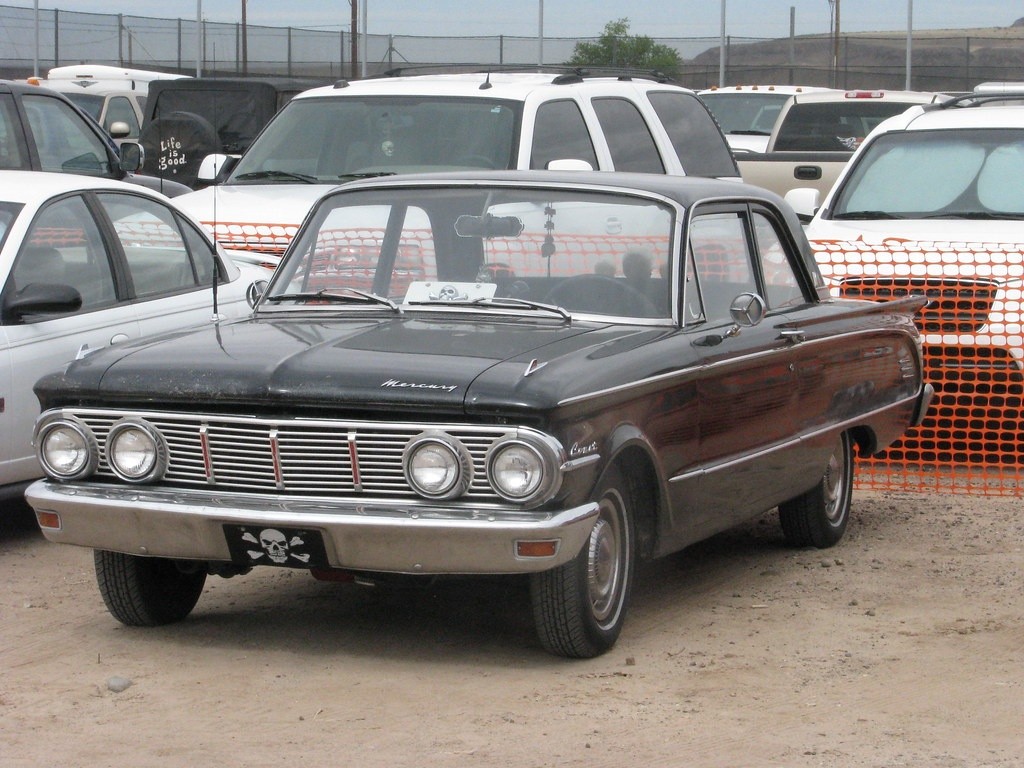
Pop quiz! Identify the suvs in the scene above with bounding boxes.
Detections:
[765,91,1024,439]
[114,70,749,282]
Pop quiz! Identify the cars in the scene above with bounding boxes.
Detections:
[695,80,1024,224]
[0,168,281,489]
[0,65,335,225]
[23,168,935,661]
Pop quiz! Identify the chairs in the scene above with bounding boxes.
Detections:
[11,245,65,292]
